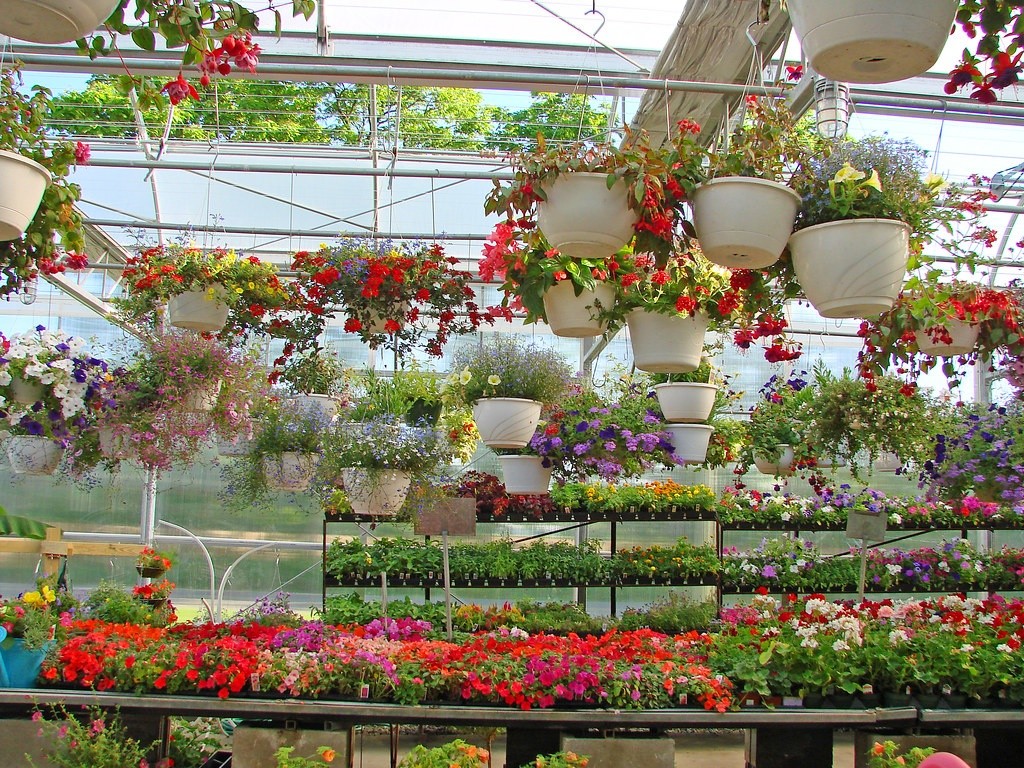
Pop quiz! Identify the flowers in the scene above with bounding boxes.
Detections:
[0,592,1024,713]
[0,0,1024,510]
[131,578,174,598]
[622,533,1024,584]
[519,750,591,768]
[24,680,174,768]
[396,739,489,768]
[273,746,343,768]
[138,545,176,569]
[864,741,936,768]
[169,716,226,767]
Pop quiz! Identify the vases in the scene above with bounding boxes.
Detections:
[816,444,844,467]
[134,565,163,578]
[217,417,266,454]
[496,454,551,496]
[133,598,166,608]
[723,582,1013,593]
[874,451,901,473]
[167,278,230,329]
[341,467,411,514]
[292,395,340,426]
[915,313,980,356]
[776,0,958,83]
[265,451,319,491]
[788,219,914,318]
[627,307,708,373]
[0,149,53,239]
[736,686,1024,715]
[348,294,408,334]
[326,511,409,522]
[663,424,714,463]
[471,398,544,450]
[622,575,718,587]
[724,519,1024,530]
[654,381,718,422]
[535,173,639,257]
[11,374,44,404]
[477,508,716,523]
[97,424,132,458]
[542,277,617,336]
[686,177,802,269]
[183,379,222,412]
[6,435,64,475]
[749,444,796,474]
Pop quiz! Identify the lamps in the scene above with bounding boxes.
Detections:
[813,72,850,137]
[19,271,38,304]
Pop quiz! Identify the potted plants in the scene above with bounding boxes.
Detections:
[327,530,621,589]
